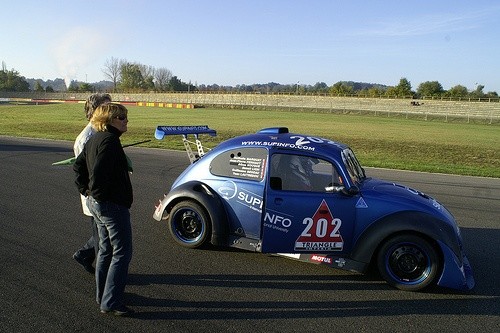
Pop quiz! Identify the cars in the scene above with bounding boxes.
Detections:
[154,125,479,293]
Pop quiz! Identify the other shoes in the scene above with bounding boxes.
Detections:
[100,306,132,316]
[73,253,95,273]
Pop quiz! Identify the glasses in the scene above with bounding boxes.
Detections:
[111,112,127,121]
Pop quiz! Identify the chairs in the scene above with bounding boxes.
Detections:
[271,155,287,190]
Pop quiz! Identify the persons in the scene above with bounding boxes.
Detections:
[74,102,136,317]
[72,92,134,275]
